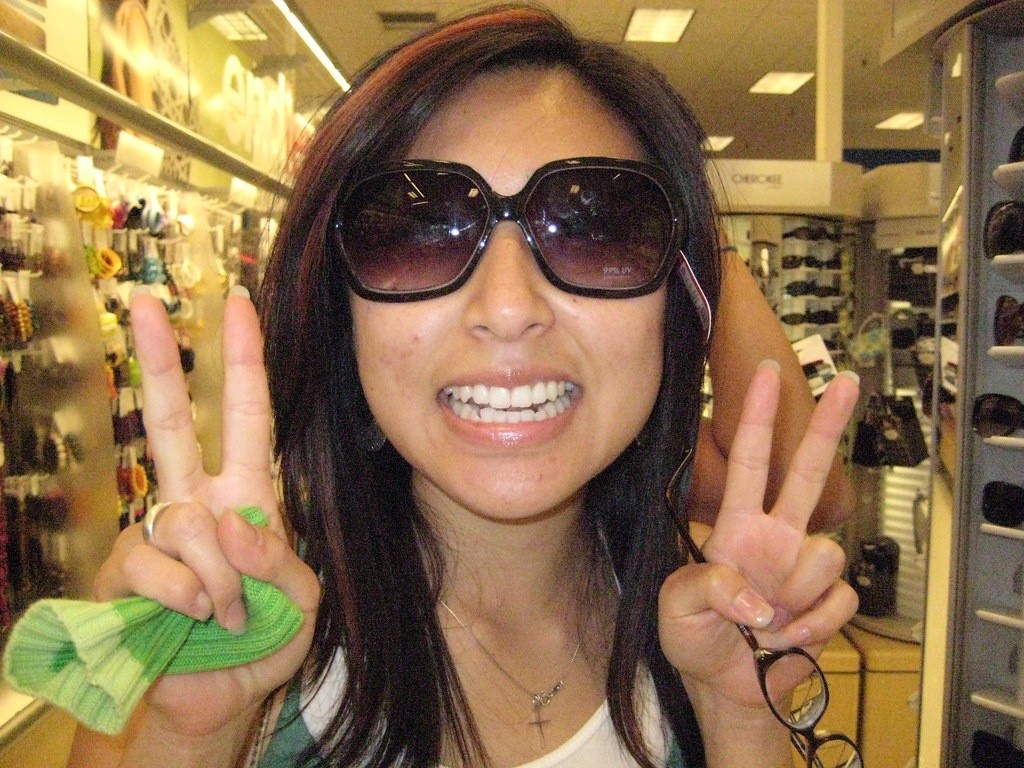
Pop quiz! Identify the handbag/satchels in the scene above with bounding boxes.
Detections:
[848,267,936,466]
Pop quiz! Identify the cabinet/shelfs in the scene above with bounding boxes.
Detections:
[0,110,1024,768]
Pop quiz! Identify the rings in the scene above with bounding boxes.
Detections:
[143,502,171,545]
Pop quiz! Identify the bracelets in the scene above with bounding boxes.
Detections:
[715,245,738,255]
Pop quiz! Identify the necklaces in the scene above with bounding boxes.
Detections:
[438,536,597,749]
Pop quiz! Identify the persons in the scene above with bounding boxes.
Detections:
[67,5,860,768]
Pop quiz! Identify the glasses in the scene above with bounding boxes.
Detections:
[960,112,1024,765]
[772,222,844,367]
[326,156,683,304]
[663,447,866,768]
